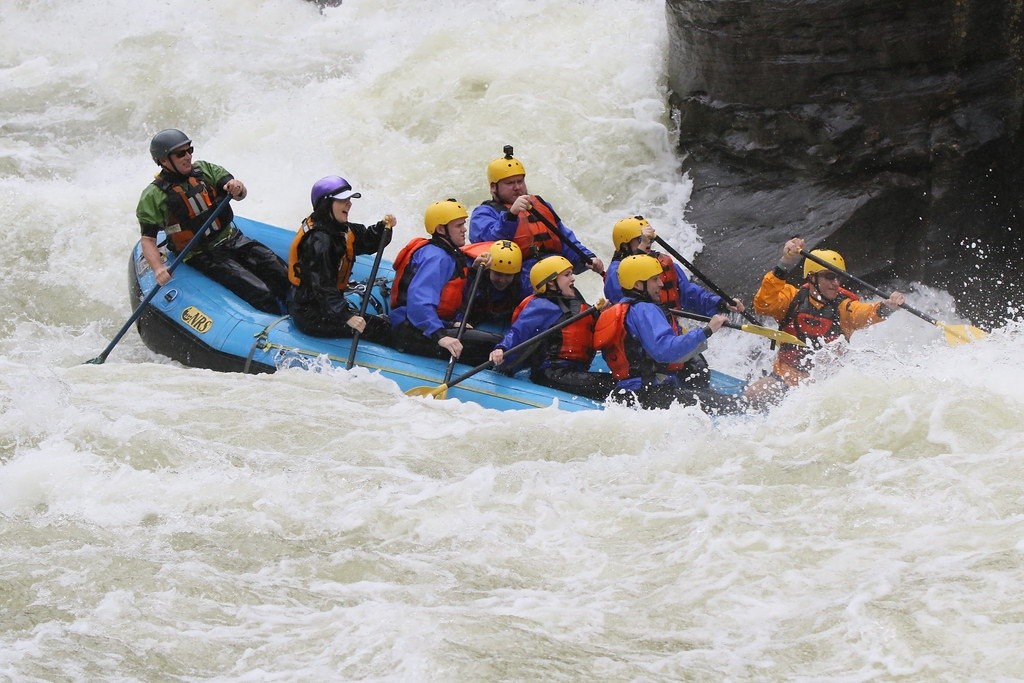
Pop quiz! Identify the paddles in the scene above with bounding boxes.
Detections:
[652,233,781,352]
[667,308,809,349]
[434,252,488,399]
[787,240,989,348]
[345,215,397,371]
[404,297,611,398]
[80,182,245,365]
[527,205,606,279]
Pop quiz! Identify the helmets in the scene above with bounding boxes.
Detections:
[612,217,656,251]
[488,240,522,274]
[803,248,846,278]
[311,175,361,210]
[150,129,192,166]
[529,255,573,293]
[424,201,468,235]
[486,156,526,185]
[617,254,664,290]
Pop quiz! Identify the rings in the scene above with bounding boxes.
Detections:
[237,186,240,188]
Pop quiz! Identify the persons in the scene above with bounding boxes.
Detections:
[390,200,501,358]
[455,241,538,328]
[604,216,745,318]
[137,129,289,310]
[596,255,737,415]
[743,238,904,405]
[489,256,615,396]
[469,156,603,297]
[288,174,431,353]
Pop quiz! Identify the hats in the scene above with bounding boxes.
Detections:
[332,190,360,201]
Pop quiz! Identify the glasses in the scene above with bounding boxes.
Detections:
[634,237,655,244]
[169,146,193,159]
[808,272,843,282]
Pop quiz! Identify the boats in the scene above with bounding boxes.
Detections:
[126,212,785,429]
